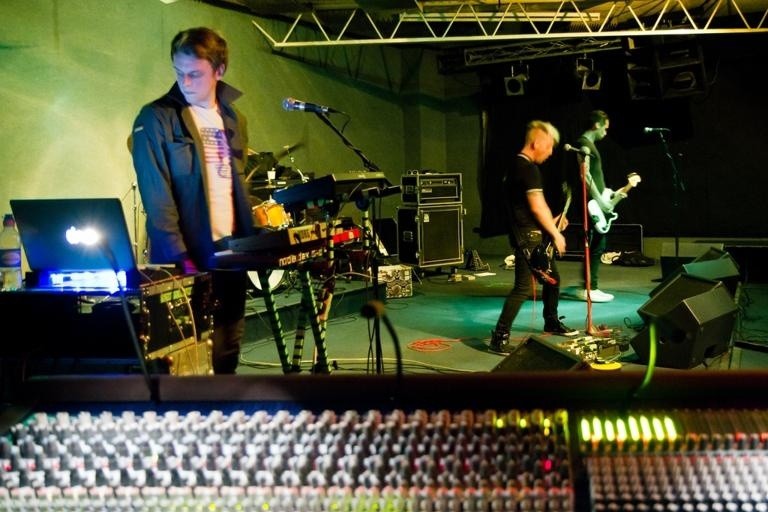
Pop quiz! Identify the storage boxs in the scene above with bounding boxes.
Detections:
[377,264,413,299]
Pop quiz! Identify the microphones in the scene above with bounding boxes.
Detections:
[282,97,337,114]
[359,300,403,376]
[564,144,594,157]
[644,127,670,134]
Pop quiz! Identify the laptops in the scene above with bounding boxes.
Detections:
[10,198,176,289]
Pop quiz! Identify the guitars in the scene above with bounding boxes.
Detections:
[531,184,573,285]
[588,161,642,234]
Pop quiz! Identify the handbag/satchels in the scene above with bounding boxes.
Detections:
[245,152,273,179]
[612,252,655,267]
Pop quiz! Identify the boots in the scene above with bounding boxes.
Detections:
[488,331,515,355]
[545,313,579,336]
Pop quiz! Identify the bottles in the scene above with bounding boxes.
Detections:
[0,214,22,292]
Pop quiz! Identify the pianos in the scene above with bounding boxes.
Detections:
[273,170,384,205]
[207,220,355,268]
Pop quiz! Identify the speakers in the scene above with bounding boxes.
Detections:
[556,223,584,262]
[637,271,728,327]
[395,205,464,268]
[626,39,706,102]
[606,224,643,256]
[488,335,593,374]
[628,286,739,369]
[660,256,696,281]
[690,247,740,270]
[648,258,740,299]
[724,246,768,284]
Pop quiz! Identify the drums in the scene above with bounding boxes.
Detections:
[246,270,288,295]
[251,197,295,230]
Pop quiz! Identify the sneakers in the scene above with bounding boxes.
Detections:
[584,289,614,302]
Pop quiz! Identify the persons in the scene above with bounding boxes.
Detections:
[129,26,259,374]
[486,120,581,356]
[572,108,630,302]
